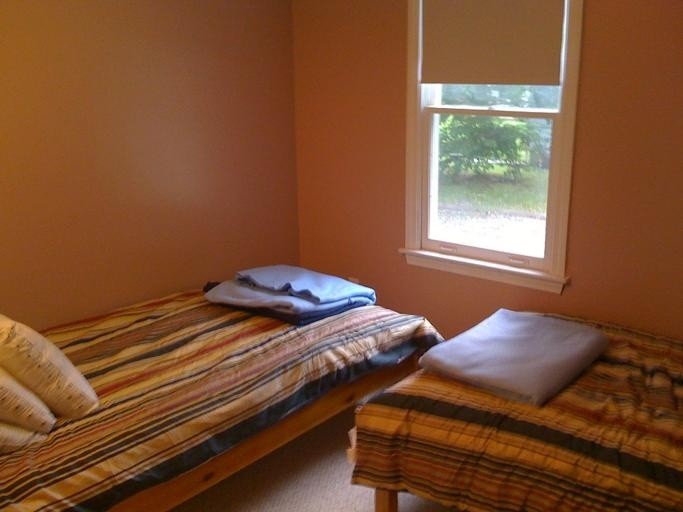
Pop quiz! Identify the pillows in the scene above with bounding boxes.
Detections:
[0,315,100,418]
[0,367,57,434]
[0,421,47,455]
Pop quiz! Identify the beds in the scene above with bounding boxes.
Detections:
[0,278,423,512]
[356,313,683,512]
